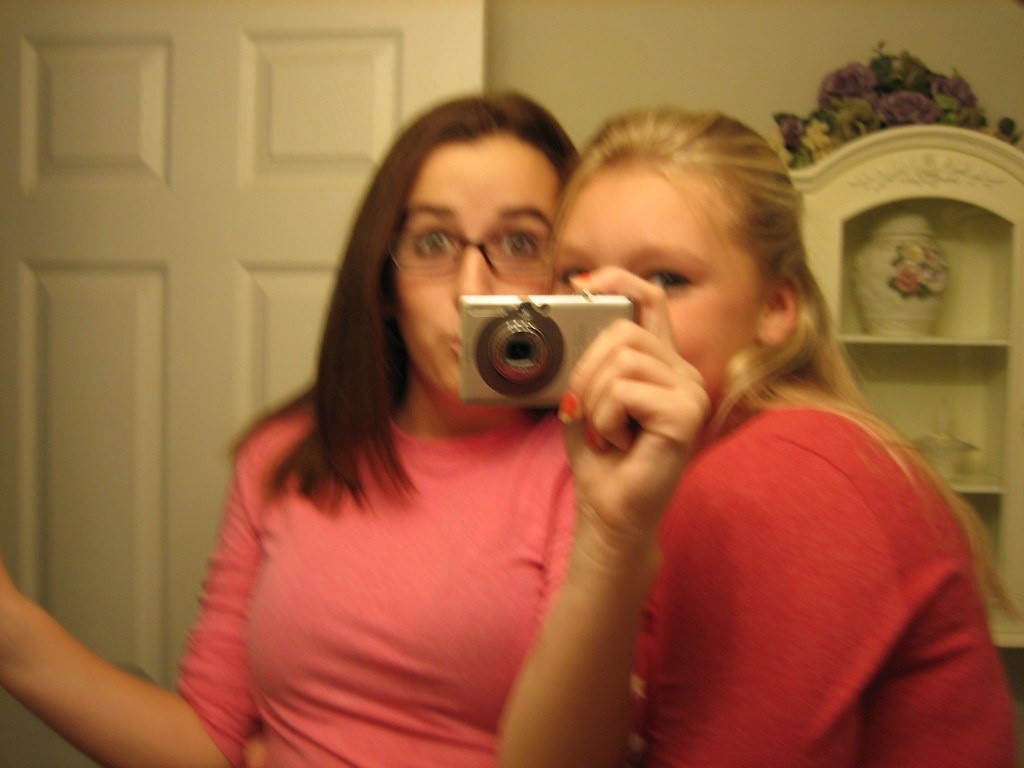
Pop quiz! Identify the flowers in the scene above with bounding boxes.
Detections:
[774,39,1023,176]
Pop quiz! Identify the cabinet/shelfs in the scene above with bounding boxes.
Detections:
[780,120,1024,651]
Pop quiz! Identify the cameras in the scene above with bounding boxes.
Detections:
[459,295,635,407]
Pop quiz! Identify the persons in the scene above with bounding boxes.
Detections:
[541,103,1024,768]
[1,90,715,767]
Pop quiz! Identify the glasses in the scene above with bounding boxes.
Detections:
[388,223,553,281]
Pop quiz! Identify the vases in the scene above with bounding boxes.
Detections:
[852,198,954,336]
